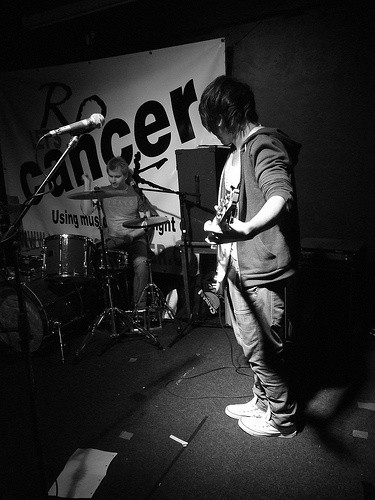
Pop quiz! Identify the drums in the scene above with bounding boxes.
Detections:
[93,247,129,273]
[0,272,85,354]
[41,233,96,283]
[17,248,44,276]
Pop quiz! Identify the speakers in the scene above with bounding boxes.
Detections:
[175,147,233,242]
[282,264,368,347]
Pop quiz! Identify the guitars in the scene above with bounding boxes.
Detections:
[202,187,240,248]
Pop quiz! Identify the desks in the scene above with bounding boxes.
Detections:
[176,241,220,321]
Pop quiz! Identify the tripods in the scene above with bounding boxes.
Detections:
[73,197,164,358]
[126,226,184,334]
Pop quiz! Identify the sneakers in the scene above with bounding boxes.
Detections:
[237,416,298,438]
[225,396,265,418]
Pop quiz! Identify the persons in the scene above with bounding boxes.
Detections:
[198,75,309,438]
[80,156,159,332]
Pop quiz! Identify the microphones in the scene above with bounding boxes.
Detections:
[47,114,105,137]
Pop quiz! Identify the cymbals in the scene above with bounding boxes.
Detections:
[66,191,138,200]
[122,216,168,229]
